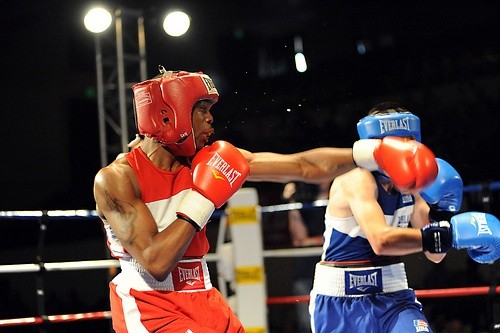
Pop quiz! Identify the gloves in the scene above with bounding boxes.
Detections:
[421,211,500,263]
[419,157,463,212]
[175,140,251,232]
[352,135,438,189]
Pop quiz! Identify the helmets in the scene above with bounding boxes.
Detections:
[357,111,421,180]
[132,69,219,157]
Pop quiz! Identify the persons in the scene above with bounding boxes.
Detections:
[283,179,333,333]
[94,64,438,333]
[278,102,500,333]
